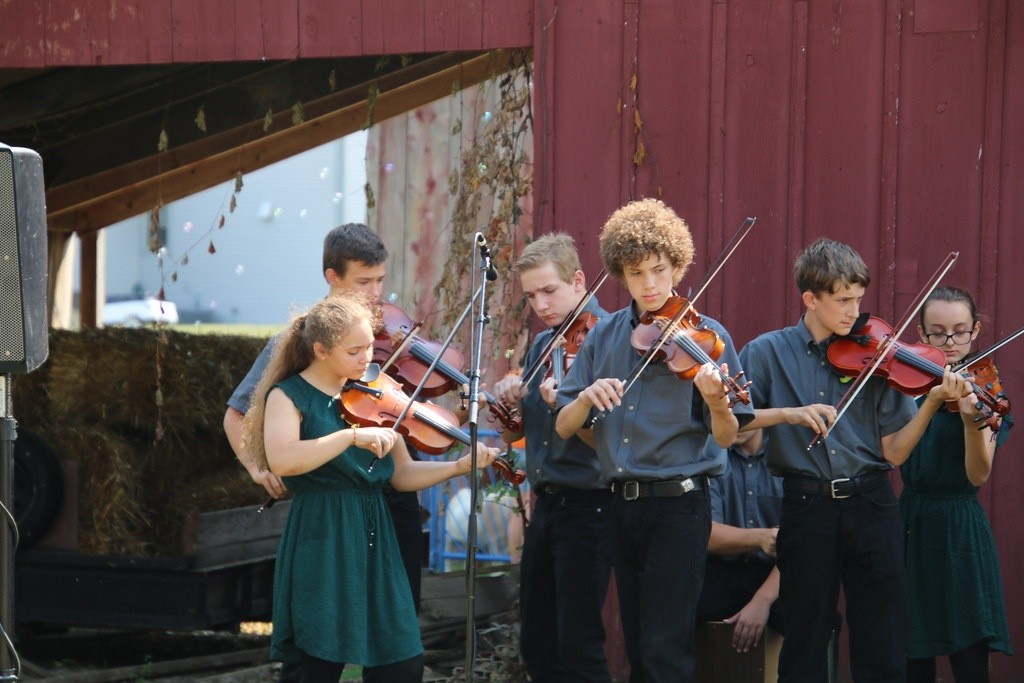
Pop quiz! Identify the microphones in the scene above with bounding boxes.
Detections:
[478,233,498,281]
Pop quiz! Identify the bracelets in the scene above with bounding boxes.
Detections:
[350,423,360,447]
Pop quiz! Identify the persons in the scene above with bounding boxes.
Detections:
[895,287,1001,683]
[554,196,756,683]
[224,223,489,683]
[240,291,500,682]
[707,427,829,682]
[492,231,616,683]
[737,240,975,683]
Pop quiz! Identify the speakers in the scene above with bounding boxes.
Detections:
[0,144,50,375]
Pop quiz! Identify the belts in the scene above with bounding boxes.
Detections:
[617,476,710,501]
[783,470,888,499]
[538,484,574,496]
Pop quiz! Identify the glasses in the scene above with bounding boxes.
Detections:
[922,321,976,347]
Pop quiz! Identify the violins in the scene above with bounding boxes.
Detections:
[944,350,1001,434]
[362,299,526,436]
[826,313,1012,418]
[627,294,756,410]
[539,310,600,409]
[339,363,527,488]
